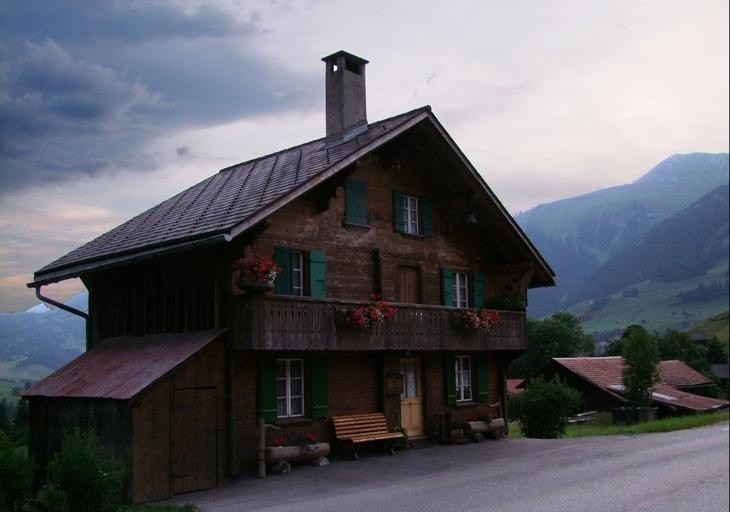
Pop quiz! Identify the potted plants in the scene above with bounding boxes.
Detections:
[611,332,661,425]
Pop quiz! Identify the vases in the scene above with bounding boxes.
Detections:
[236,277,275,294]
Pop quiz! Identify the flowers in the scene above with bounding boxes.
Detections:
[464,308,504,334]
[233,255,284,281]
[466,412,497,423]
[271,433,317,446]
[346,291,396,329]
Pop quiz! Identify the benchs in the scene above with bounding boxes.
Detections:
[330,412,405,460]
